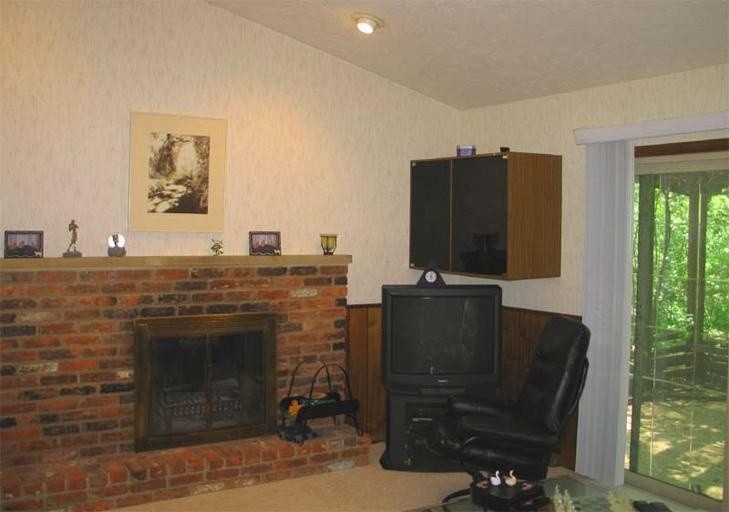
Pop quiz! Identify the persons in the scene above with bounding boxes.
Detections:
[67,220,79,252]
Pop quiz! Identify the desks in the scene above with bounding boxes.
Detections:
[422,471,669,511]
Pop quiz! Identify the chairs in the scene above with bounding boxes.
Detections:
[425,315,592,502]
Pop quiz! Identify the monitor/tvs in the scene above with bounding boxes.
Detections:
[380,285,502,395]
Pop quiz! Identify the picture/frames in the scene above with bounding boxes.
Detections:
[248,231,282,256]
[3,229,45,259]
[126,111,229,234]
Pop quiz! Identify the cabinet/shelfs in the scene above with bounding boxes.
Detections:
[407,151,564,282]
[379,392,470,472]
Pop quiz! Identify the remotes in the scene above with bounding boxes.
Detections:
[633,501,651,512]
[650,502,671,512]
